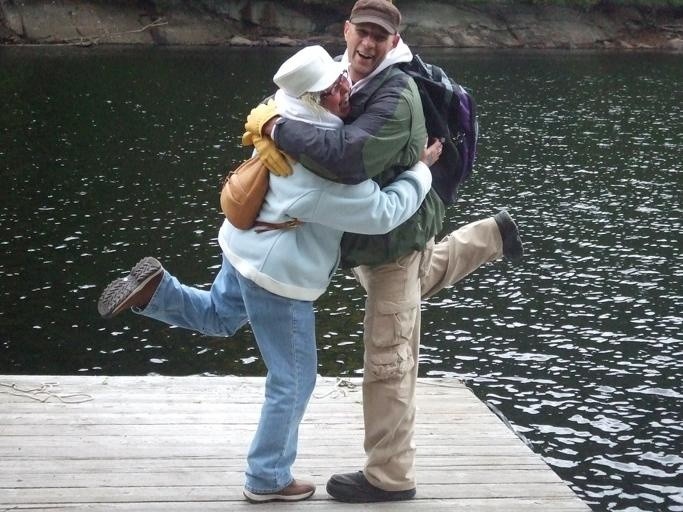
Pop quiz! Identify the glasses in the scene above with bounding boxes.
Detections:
[321,69,348,98]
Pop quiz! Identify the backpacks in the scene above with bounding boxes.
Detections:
[220,155,303,233]
[401,53,480,205]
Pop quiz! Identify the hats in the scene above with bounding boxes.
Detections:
[273,45,349,97]
[349,0,402,35]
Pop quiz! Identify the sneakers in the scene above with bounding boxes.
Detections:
[494,210,524,266]
[243,477,316,504]
[326,471,417,503]
[98,257,164,321]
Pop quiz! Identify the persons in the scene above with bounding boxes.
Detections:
[242,0,525,502]
[97,44,443,505]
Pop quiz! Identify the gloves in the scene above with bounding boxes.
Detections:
[245,99,280,137]
[241,131,295,178]
